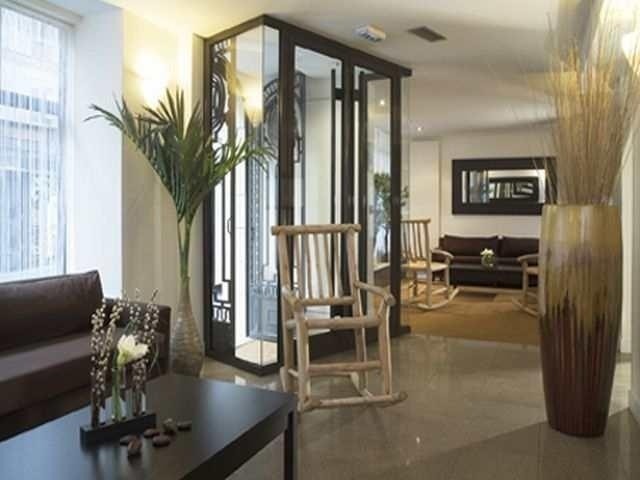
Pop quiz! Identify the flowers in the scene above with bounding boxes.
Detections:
[112,333,148,420]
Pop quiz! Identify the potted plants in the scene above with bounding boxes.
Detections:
[85,86,279,380]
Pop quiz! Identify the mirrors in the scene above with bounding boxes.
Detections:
[450,157,557,215]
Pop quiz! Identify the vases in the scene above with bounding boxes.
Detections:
[537,203,628,441]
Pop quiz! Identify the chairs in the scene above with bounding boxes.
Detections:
[269,221,407,411]
[511,252,538,316]
[394,216,463,311]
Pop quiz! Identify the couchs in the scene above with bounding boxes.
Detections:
[1,269,171,441]
[431,234,538,291]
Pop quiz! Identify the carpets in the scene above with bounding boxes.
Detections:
[373,275,541,347]
